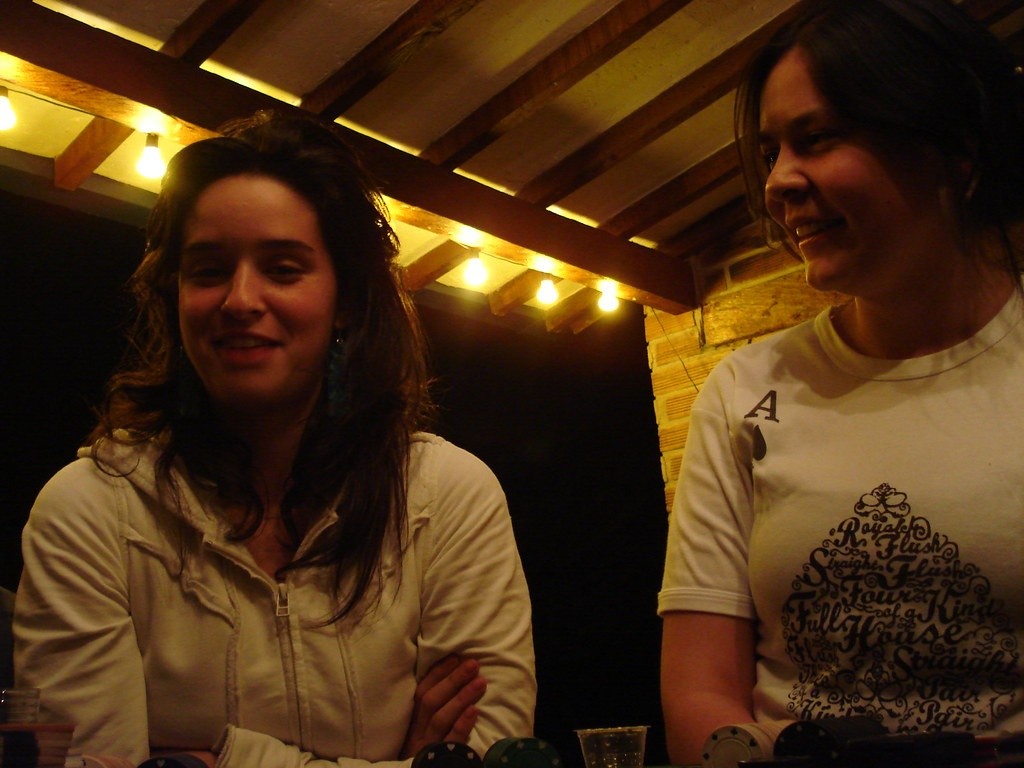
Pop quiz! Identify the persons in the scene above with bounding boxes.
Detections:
[655,0,1024,768]
[12,113,540,768]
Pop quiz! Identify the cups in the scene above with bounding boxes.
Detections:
[575,726,651,768]
